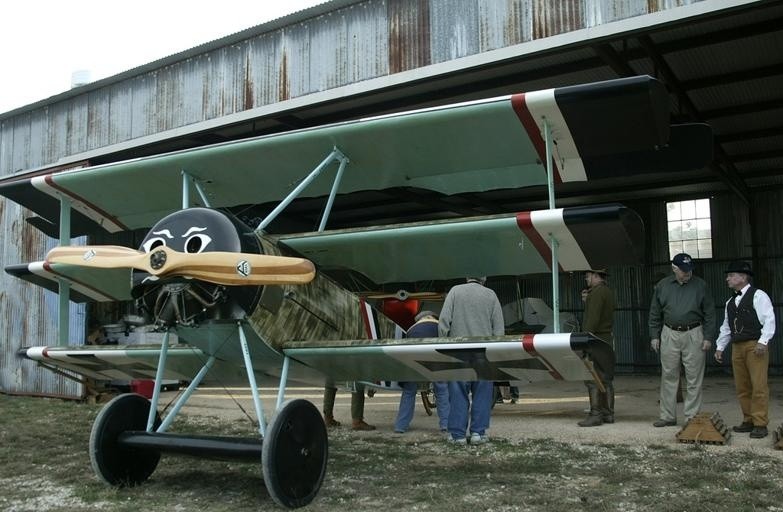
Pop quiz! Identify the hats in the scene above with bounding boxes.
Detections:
[672,253,694,273]
[587,263,610,275]
[412,310,439,321]
[721,261,755,278]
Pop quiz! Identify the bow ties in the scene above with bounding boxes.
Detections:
[732,290,743,299]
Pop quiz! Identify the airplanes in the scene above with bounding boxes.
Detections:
[1,74,670,512]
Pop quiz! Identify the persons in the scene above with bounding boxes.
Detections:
[390,310,449,436]
[576,263,617,428]
[435,275,504,447]
[321,381,377,431]
[711,260,777,438]
[647,252,716,428]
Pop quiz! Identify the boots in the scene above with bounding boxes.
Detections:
[577,379,615,427]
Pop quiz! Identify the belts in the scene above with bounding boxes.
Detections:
[661,321,702,331]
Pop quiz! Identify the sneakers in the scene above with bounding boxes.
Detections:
[732,420,753,432]
[749,425,769,439]
[445,431,491,446]
[653,419,677,427]
